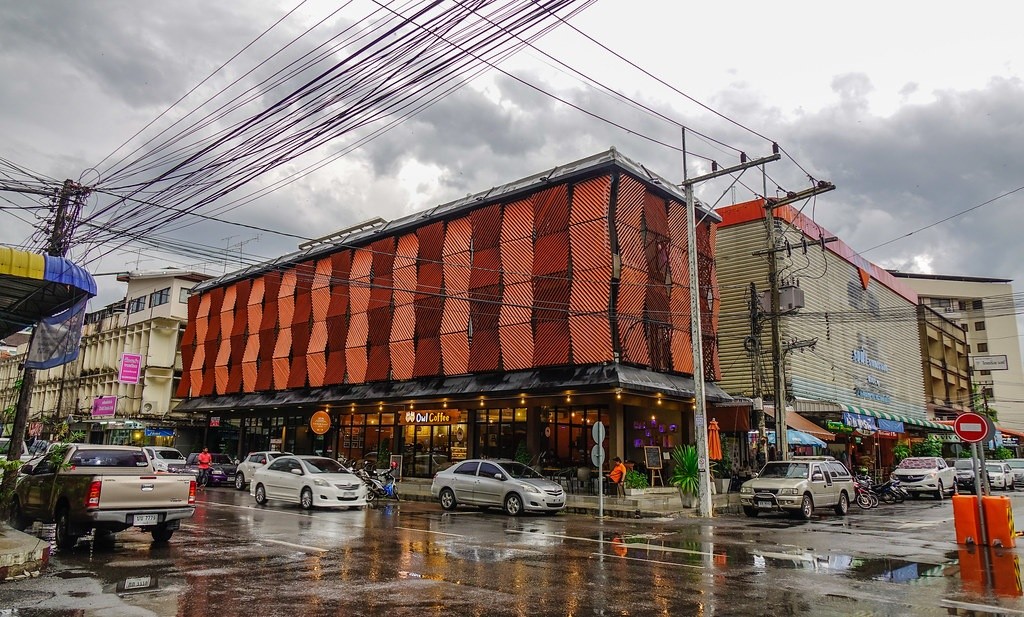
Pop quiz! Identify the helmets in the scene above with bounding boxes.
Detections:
[391,461,398,468]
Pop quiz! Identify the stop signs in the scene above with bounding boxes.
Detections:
[953,412,990,443]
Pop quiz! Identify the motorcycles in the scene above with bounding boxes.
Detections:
[848,468,909,509]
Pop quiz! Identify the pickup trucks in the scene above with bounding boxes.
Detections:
[889,456,959,500]
[6,444,198,547]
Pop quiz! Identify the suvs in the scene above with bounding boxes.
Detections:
[144,446,237,487]
[739,456,855,520]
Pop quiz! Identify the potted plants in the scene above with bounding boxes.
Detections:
[374,437,392,485]
[624,468,652,496]
[667,444,719,508]
[715,432,732,494]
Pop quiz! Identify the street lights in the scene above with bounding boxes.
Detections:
[6,269,192,459]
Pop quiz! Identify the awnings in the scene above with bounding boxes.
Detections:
[764,408,835,440]
[795,401,954,431]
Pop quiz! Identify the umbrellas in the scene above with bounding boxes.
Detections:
[767,430,826,448]
[708,418,722,477]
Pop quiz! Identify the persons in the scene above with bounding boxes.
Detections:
[198,447,213,488]
[604,457,626,496]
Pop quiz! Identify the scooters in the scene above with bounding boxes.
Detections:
[341,459,399,505]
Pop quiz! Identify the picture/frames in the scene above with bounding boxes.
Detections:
[344,427,364,450]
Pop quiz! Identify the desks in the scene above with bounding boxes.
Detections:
[544,468,610,481]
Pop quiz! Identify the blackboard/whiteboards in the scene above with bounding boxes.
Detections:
[644,446,662,469]
[390,455,402,479]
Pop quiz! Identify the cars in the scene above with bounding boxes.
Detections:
[0,436,70,488]
[945,458,1024,495]
[235,451,295,491]
[250,455,368,510]
[431,459,567,516]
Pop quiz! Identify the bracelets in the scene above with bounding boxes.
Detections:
[621,480,624,482]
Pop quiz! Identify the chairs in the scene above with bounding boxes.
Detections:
[553,467,626,499]
